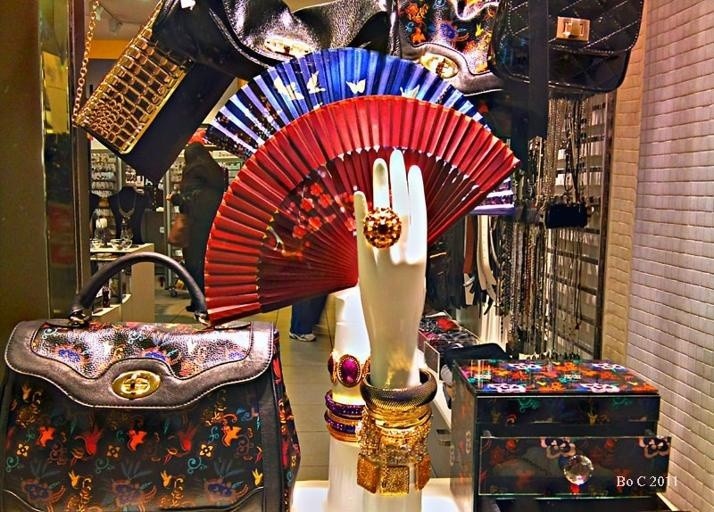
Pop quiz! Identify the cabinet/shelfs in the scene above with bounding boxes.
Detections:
[88,147,174,296]
[446,352,672,512]
[164,139,247,295]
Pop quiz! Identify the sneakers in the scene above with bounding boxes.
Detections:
[289,332,315,342]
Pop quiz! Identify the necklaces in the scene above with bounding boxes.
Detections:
[497,95,592,360]
[117,191,138,220]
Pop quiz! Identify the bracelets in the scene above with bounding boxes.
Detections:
[356,357,437,497]
[324,352,371,443]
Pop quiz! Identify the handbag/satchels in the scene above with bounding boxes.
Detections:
[72,2,645,230]
[0,252,301,512]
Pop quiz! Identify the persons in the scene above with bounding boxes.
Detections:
[353,148,437,512]
[288,294,328,342]
[325,277,370,512]
[107,184,147,245]
[166,142,227,313]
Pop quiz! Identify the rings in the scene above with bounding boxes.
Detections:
[362,207,402,249]
